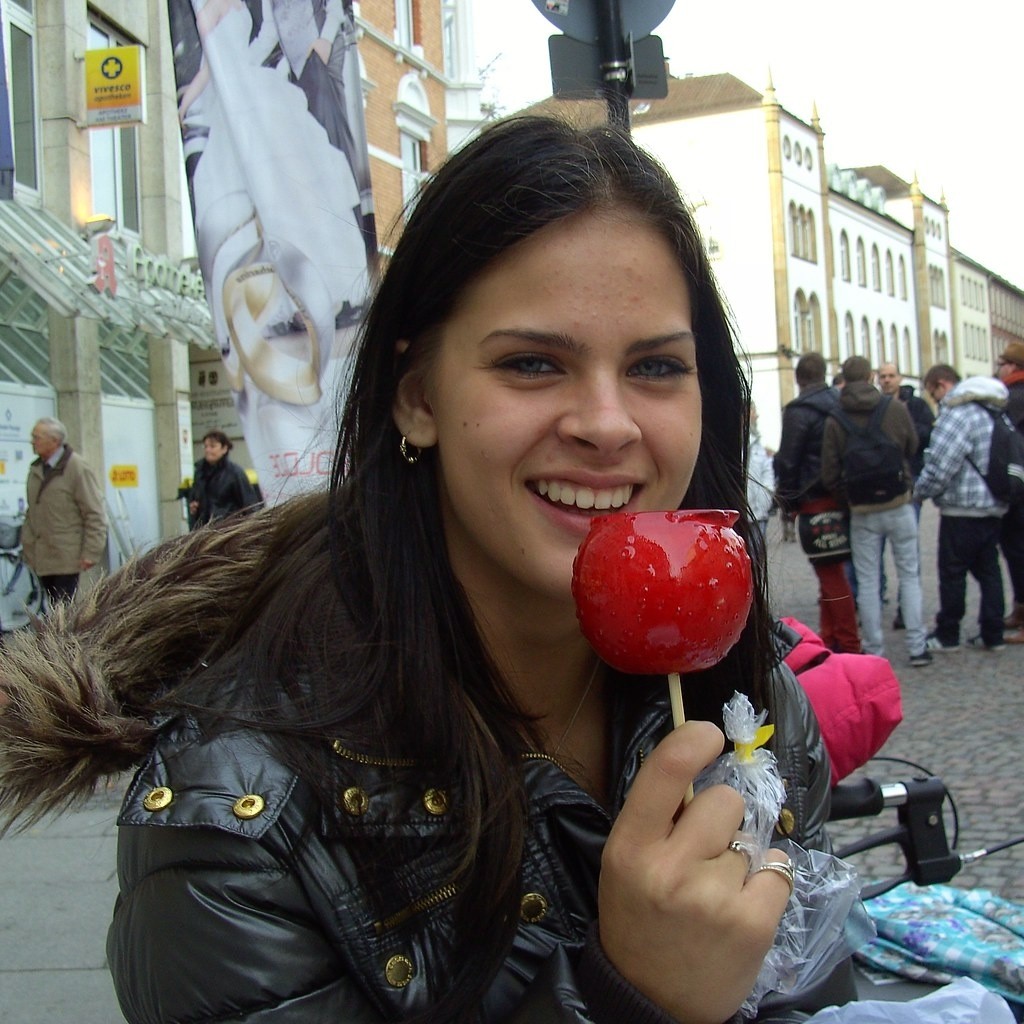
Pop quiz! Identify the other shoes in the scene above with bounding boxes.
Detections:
[925,634,959,652]
[965,633,989,648]
[1003,601,1024,627]
[893,616,906,629]
[910,652,931,665]
[1002,630,1024,643]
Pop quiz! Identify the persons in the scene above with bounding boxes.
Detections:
[1,118,873,1024]
[817,355,937,667]
[913,363,1007,650]
[189,428,259,531]
[746,402,776,555]
[775,354,866,655]
[994,339,1024,644]
[19,417,107,608]
[878,361,935,629]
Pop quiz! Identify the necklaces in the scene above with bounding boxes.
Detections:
[513,653,602,759]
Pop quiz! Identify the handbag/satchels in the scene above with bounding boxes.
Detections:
[798,500,851,564]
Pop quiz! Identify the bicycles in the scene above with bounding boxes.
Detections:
[0,507,54,633]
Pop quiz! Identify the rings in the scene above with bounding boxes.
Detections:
[729,841,753,865]
[743,859,797,894]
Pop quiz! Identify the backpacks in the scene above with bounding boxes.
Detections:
[966,401,1024,505]
[830,395,907,504]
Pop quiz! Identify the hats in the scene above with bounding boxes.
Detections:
[796,353,826,385]
[843,356,870,381]
[999,343,1024,368]
